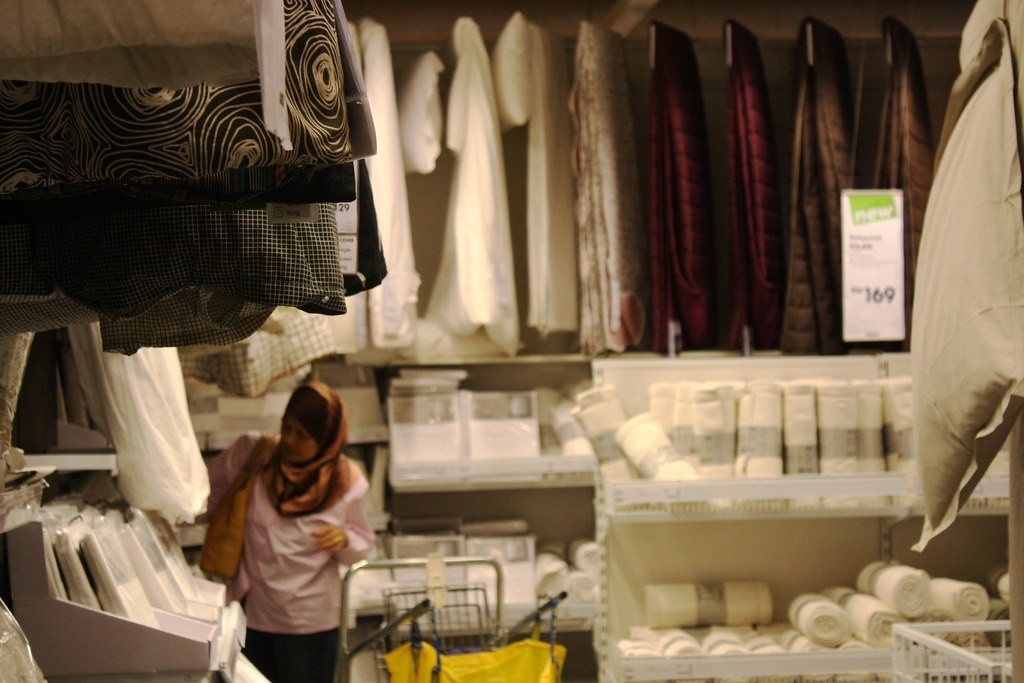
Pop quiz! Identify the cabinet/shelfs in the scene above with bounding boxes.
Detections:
[0,295,1012,683]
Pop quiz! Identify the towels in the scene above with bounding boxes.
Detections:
[534,536,600,604]
[545,375,918,504]
[619,559,1011,682]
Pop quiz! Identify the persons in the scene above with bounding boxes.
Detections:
[176,381,376,683]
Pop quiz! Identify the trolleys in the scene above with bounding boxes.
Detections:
[332,557,568,683]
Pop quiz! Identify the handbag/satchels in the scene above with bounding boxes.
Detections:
[200,436,274,578]
